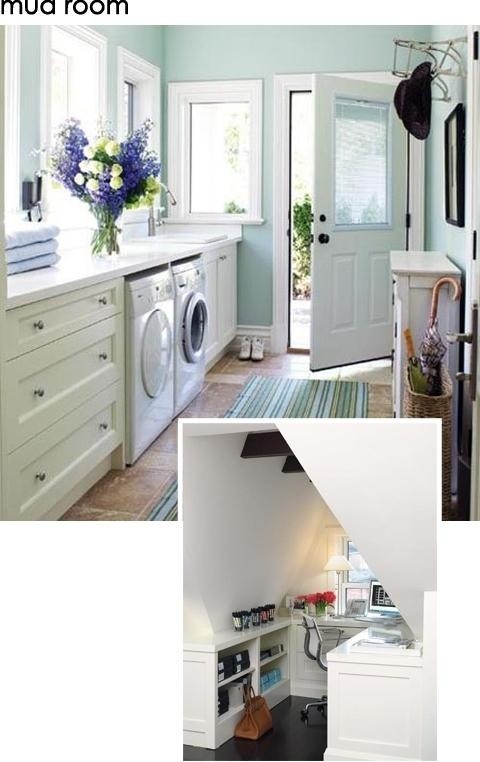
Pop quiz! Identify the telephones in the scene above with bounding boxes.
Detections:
[342,597,368,618]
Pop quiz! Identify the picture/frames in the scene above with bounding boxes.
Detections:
[443,103,465,228]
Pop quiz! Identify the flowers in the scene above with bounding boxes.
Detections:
[28,117,164,253]
[297,591,334,614]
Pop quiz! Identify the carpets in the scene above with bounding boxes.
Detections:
[134,372,369,522]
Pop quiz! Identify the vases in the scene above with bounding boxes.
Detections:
[308,605,327,617]
[86,209,126,255]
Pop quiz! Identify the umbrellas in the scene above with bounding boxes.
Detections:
[419,276,459,394]
[404,328,425,394]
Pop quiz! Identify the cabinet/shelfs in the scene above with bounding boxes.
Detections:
[157,222,244,372]
[183,618,290,749]
[2,245,127,521]
[290,609,387,700]
[323,627,437,761]
[389,250,464,420]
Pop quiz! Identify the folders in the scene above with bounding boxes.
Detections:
[235,659,250,674]
[218,655,234,673]
[235,649,249,666]
[219,665,234,683]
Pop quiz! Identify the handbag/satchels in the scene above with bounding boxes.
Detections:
[234,683,273,740]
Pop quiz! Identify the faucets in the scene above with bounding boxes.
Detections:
[148,181,176,235]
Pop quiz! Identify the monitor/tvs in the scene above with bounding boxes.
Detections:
[368,580,399,612]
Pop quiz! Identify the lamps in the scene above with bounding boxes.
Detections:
[324,556,352,619]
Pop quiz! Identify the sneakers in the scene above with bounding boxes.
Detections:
[238,335,252,361]
[251,336,265,361]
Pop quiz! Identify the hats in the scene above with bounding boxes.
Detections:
[393,61,432,141]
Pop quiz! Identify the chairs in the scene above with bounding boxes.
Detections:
[297,615,355,728]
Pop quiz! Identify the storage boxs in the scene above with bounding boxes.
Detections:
[402,356,454,516]
